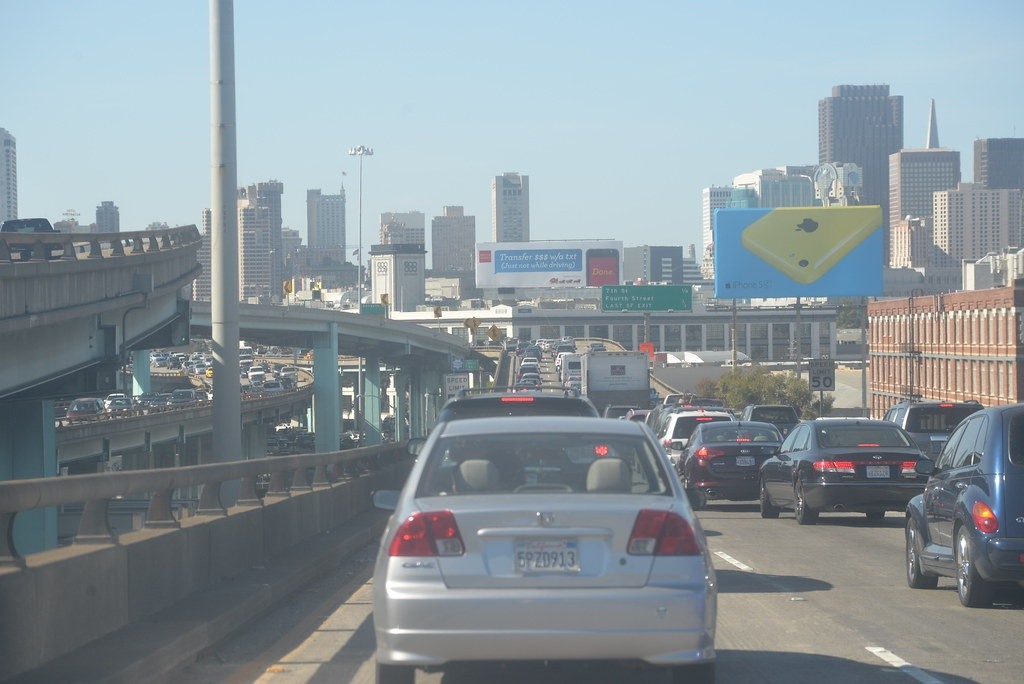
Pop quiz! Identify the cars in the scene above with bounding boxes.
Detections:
[758,416,936,524]
[255,423,394,492]
[476,336,743,475]
[130,342,300,414]
[670,420,790,509]
[904,400,1024,608]
[366,414,720,683]
[104,393,126,411]
[410,388,632,504]
[64,396,109,424]
[105,397,135,416]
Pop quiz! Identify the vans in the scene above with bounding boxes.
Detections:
[739,403,803,443]
[880,398,985,464]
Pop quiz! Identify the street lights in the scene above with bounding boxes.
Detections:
[347,144,375,308]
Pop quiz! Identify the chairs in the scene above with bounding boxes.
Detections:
[452,459,502,495]
[715,435,725,441]
[586,457,631,493]
[753,436,770,442]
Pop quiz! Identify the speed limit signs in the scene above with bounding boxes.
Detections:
[808,355,836,393]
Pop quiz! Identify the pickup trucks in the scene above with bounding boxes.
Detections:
[0,218,64,260]
